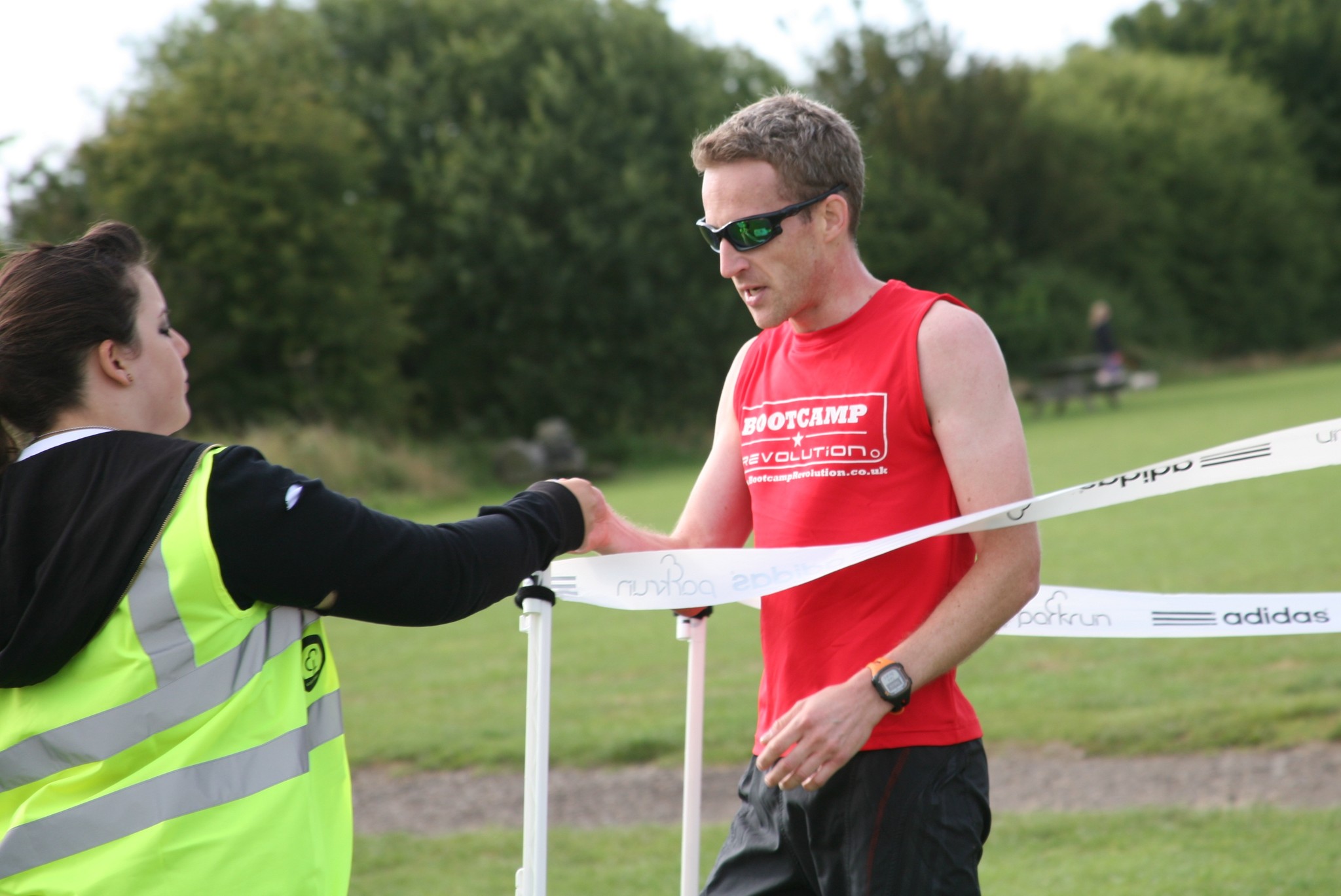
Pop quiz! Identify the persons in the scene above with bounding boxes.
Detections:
[0,220,595,896]
[566,90,1041,896]
[1089,300,1125,388]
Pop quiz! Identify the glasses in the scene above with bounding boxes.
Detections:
[693,182,852,251]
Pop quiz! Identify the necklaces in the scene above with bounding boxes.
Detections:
[30,426,118,440]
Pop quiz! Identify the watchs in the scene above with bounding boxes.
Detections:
[867,657,913,713]
